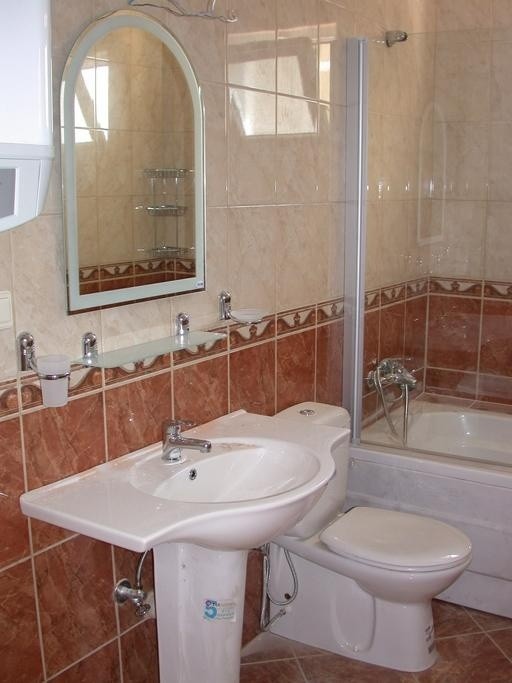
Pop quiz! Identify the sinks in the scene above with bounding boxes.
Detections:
[20,409,350,552]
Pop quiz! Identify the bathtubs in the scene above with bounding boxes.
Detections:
[343,393,512,619]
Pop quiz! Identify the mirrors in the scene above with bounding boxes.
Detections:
[59,10,208,316]
[338,24,512,621]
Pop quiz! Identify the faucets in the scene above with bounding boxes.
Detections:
[160,420,212,461]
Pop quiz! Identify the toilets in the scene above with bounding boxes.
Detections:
[265,401,474,671]
